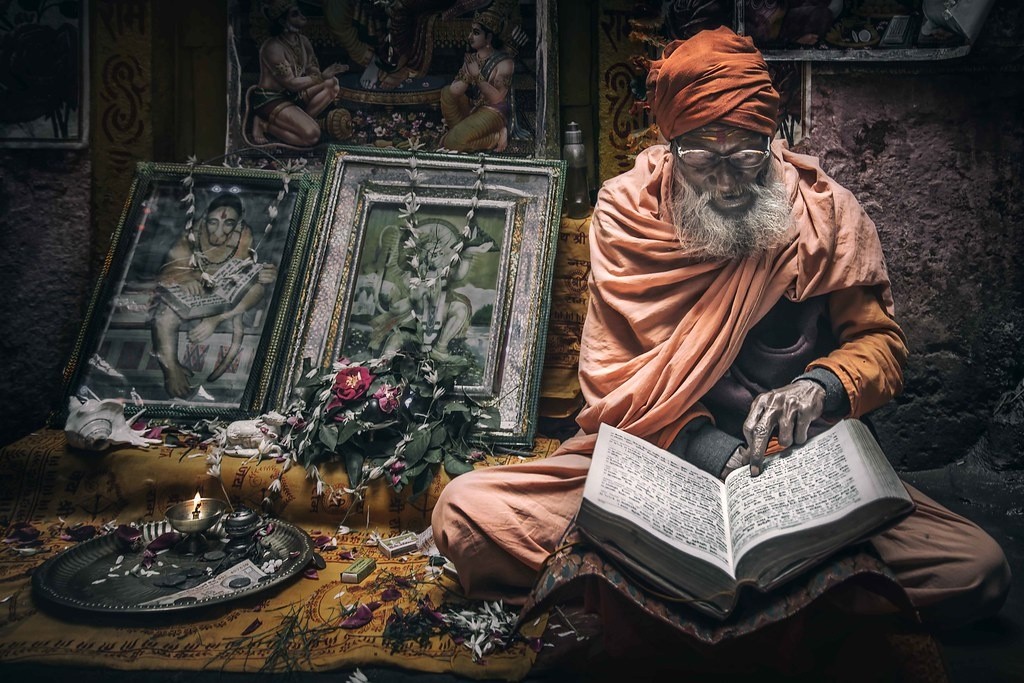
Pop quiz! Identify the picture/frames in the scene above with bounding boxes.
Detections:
[41,161,325,434]
[221,0,562,169]
[260,141,567,447]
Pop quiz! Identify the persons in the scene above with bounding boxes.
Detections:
[430,20,1013,632]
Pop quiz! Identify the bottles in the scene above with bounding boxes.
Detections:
[564,121,590,219]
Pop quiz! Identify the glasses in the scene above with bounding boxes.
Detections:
[673,136,771,168]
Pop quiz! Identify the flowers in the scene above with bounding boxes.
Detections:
[280,350,481,494]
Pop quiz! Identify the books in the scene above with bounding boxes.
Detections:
[574,415,916,619]
